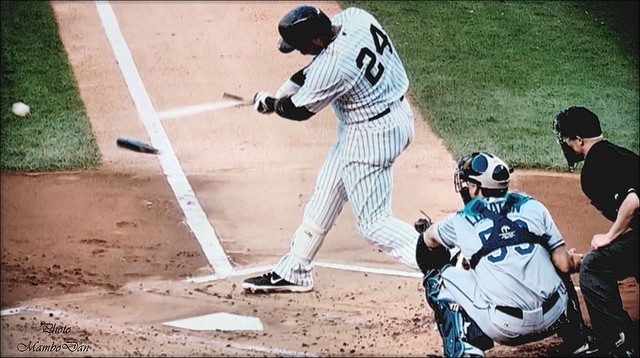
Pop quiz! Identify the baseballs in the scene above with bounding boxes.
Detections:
[12,101,30,117]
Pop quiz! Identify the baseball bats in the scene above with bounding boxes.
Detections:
[136,97,260,124]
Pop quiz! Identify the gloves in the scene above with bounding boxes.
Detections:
[254,91,276,114]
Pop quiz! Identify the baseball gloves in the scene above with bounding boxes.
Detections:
[415,219,451,274]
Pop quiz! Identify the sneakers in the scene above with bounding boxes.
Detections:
[242,272,313,295]
[571,338,638,356]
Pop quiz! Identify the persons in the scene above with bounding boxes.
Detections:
[414,150,598,358]
[242,5,470,292]
[552,107,639,358]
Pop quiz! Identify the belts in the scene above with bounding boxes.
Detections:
[496,290,560,319]
[368,95,404,121]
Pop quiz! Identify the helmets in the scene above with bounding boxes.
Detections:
[277,5,331,53]
[454,152,512,198]
[552,106,603,173]
